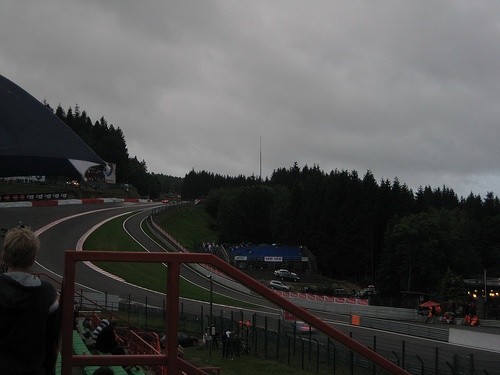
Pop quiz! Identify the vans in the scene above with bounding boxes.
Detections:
[334,289,347,297]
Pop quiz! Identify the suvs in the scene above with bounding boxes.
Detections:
[160,332,198,349]
[268,280,289,292]
[274,269,300,282]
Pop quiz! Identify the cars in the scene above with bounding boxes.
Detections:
[170,200,176,204]
[281,321,318,335]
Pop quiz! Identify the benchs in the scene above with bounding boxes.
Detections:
[55,318,147,375]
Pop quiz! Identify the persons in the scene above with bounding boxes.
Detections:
[425,306,481,327]
[72,300,252,361]
[0,225,61,375]
